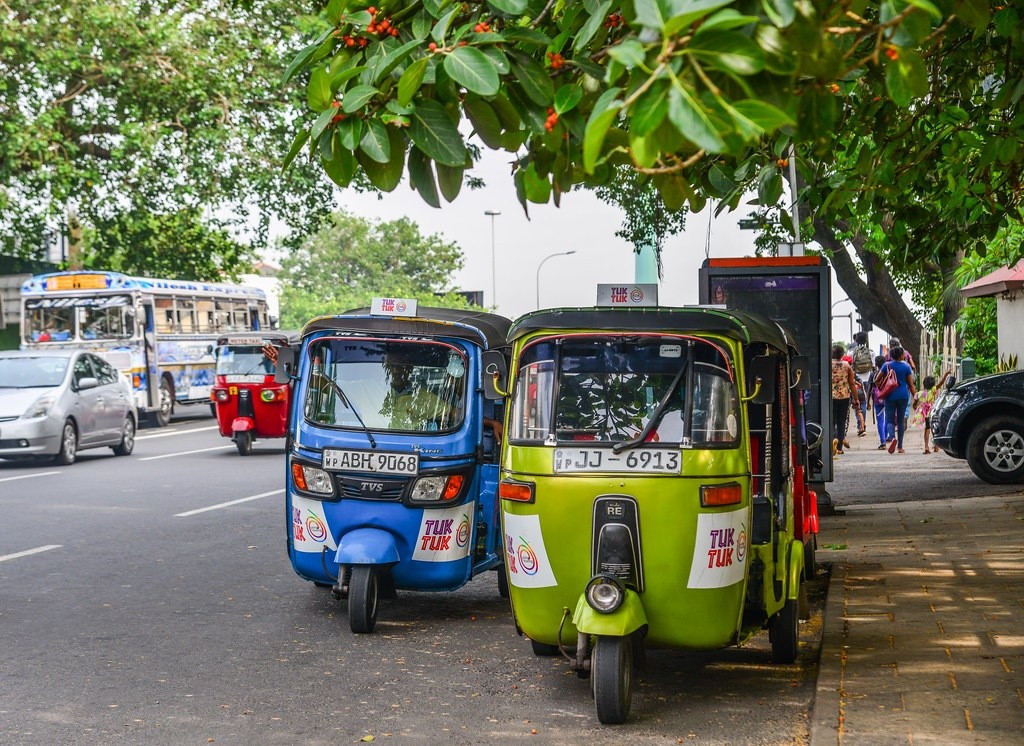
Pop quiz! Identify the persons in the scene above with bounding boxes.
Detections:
[255,342,506,446]
[827,331,952,455]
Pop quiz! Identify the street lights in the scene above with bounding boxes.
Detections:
[483,210,503,311]
[535,250,576,311]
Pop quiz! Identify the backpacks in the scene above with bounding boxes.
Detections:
[853,345,872,373]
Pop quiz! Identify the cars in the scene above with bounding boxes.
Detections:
[928,365,1024,487]
[0,350,138,465]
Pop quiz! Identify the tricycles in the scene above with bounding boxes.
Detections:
[208,328,302,456]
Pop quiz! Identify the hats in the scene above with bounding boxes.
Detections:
[842,356,852,364]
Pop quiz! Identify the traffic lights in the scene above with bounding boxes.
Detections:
[855,308,873,332]
[268,315,278,330]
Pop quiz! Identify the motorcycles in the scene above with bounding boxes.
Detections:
[19,270,270,428]
[272,297,513,632]
[501,283,809,725]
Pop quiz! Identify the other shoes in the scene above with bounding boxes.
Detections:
[888,439,905,454]
[857,425,867,437]
[832,438,844,455]
[878,443,886,450]
[842,439,850,449]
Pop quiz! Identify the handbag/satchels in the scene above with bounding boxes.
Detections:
[877,361,899,399]
